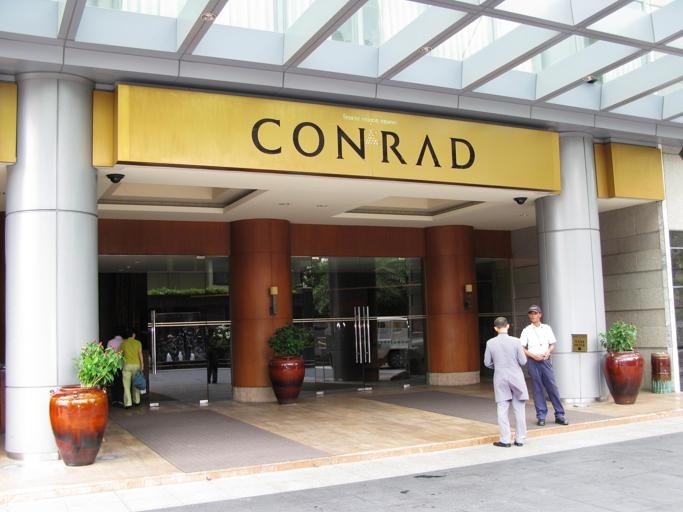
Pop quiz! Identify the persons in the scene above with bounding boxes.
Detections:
[116,327,144,409]
[106,327,126,408]
[206,337,220,384]
[483,316,530,448]
[519,304,568,426]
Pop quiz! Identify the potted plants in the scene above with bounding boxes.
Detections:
[268,318,315,405]
[599,320,645,404]
[49,338,128,466]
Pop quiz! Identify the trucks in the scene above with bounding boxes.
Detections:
[377,314,410,370]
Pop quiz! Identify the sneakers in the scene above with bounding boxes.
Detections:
[537,419,545,426]
[493,442,511,448]
[513,440,523,447]
[555,417,569,425]
[112,400,141,410]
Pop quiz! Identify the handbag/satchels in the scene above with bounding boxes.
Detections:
[132,369,147,391]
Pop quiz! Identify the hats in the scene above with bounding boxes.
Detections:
[527,304,543,313]
